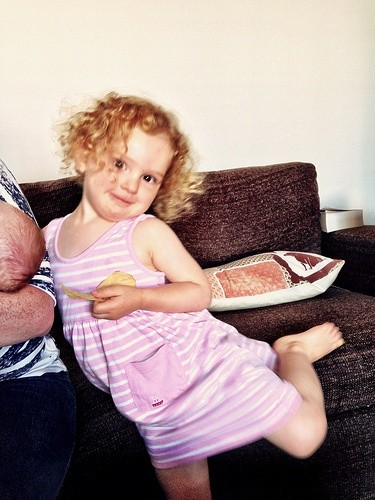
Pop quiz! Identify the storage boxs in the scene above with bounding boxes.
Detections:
[320,207,364,233]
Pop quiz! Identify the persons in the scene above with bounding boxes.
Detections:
[38,93,343,500]
[0,157,79,500]
[0,199,45,293]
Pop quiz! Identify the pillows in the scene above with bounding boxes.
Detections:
[203,250,345,312]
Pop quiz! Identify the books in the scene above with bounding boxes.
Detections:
[320,207,365,233]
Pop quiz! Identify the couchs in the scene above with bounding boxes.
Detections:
[18,161,375,500]
